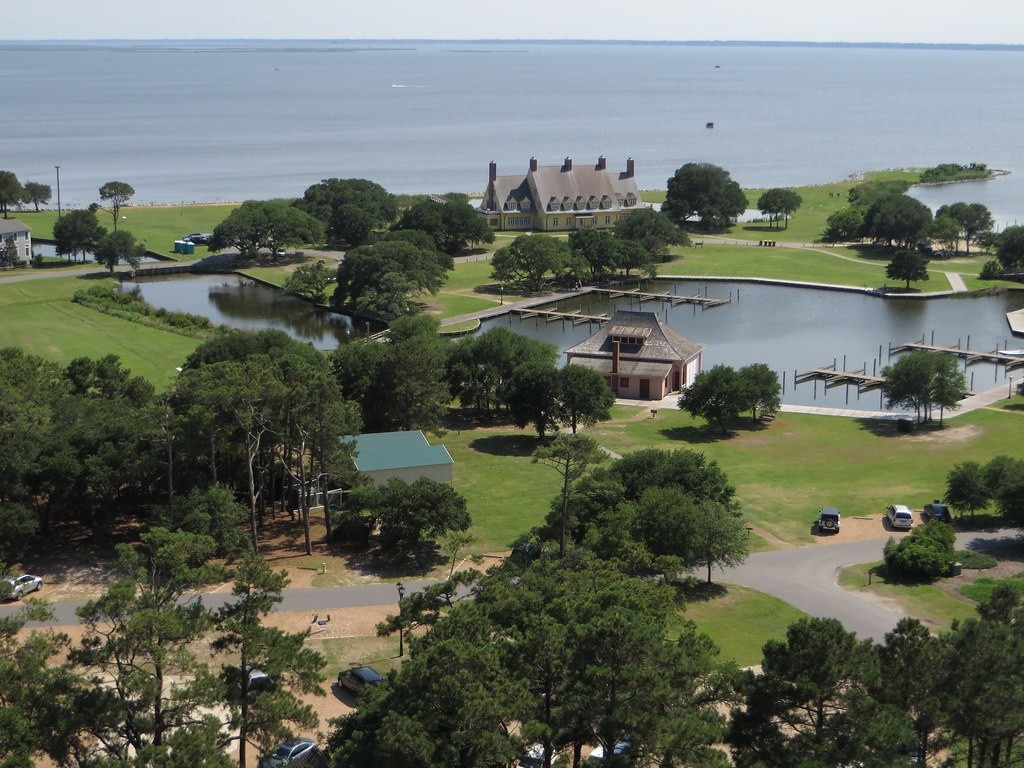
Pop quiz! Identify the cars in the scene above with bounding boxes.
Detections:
[924,502,952,524]
[183,232,210,244]
[277,248,287,256]
[257,737,317,768]
[228,664,274,693]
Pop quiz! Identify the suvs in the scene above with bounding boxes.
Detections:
[5,574,44,601]
[338,667,387,697]
[885,504,913,528]
[819,509,840,534]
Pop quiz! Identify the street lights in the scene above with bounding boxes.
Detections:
[1008,376,1013,398]
[396,581,405,656]
[55,166,61,219]
